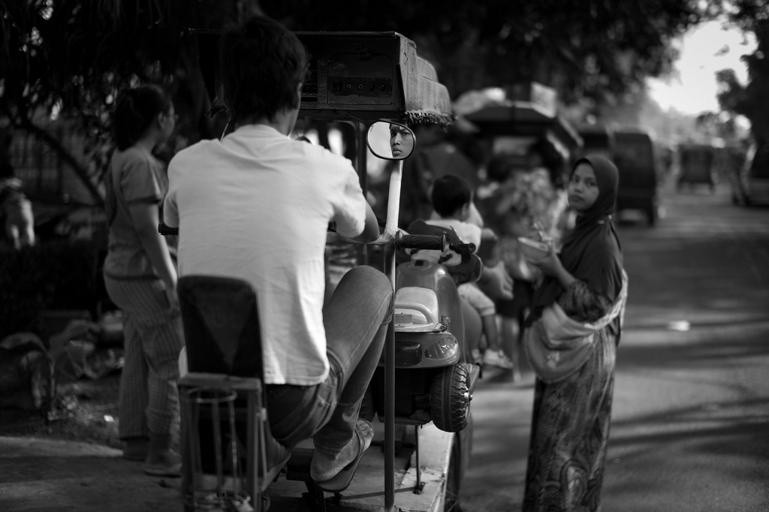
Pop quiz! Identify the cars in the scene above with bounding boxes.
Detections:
[0,113,178,337]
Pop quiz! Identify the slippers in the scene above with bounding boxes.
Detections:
[122,447,148,462]
[314,415,375,493]
[141,454,184,477]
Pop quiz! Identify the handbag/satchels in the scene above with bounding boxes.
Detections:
[521,301,602,385]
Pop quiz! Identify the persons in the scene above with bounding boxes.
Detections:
[518,149,631,511]
[485,136,567,384]
[100,83,185,479]
[156,11,396,494]
[389,122,412,157]
[423,174,517,372]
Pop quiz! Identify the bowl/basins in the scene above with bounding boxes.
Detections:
[519,236,560,261]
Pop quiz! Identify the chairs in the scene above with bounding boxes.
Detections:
[177,278,301,506]
